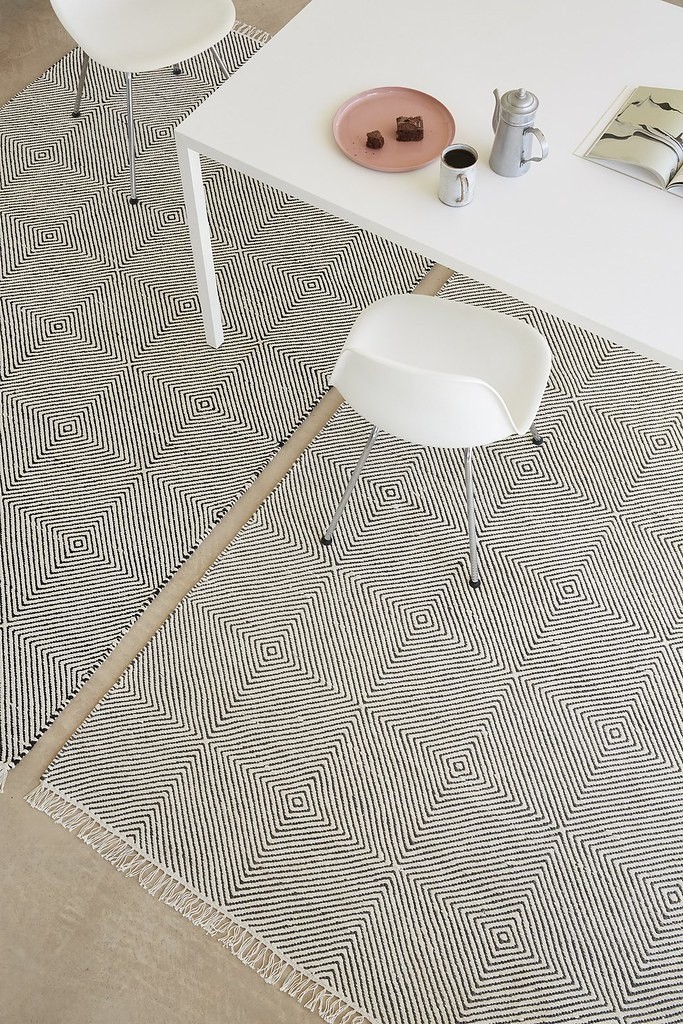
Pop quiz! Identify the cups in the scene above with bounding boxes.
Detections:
[438,143,479,206]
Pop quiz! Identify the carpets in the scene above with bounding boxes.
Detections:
[23,272,683,1024]
[0,19,436,790]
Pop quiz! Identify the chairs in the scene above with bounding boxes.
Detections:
[49,0,235,206]
[320,294,552,589]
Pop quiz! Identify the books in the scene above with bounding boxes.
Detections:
[575,85,683,197]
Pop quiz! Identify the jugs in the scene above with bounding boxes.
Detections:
[489,88,549,177]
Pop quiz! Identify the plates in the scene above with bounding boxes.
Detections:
[332,86,455,172]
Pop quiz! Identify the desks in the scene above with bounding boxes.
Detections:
[173,0,683,373]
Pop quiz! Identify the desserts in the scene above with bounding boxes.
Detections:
[365,130,385,149]
[396,115,424,141]
